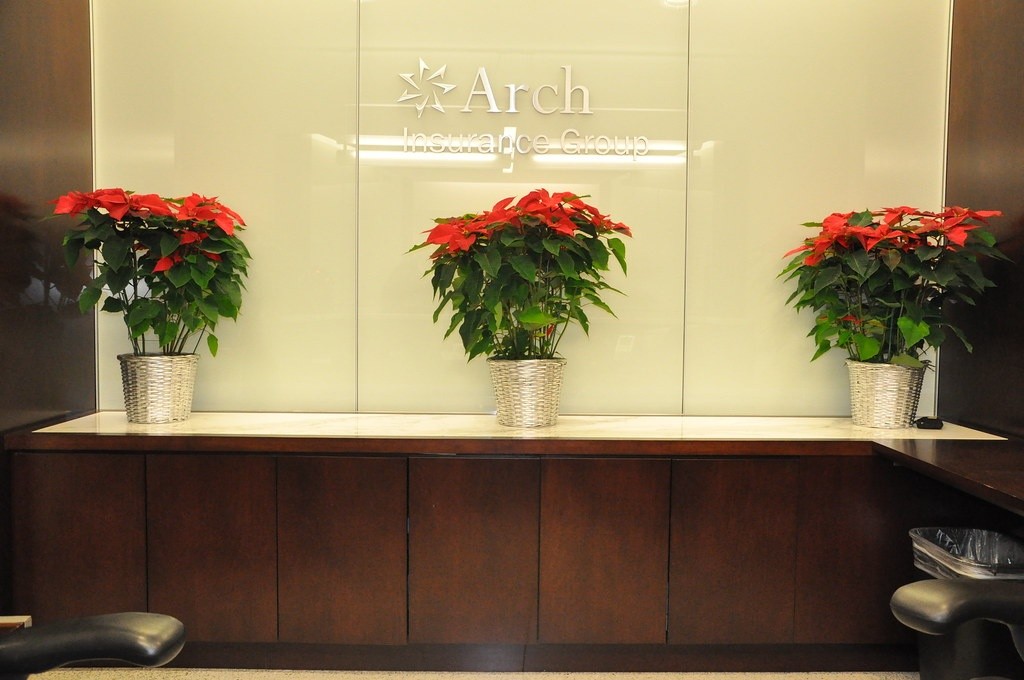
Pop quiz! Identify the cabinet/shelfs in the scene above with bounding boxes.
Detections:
[0,408,1022,672]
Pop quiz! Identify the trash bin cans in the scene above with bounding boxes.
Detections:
[909,527,1024,680]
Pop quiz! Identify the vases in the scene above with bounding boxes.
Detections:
[843,358,930,427]
[487,357,566,427]
[116,352,200,425]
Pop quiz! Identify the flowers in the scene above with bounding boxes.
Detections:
[775,207,1012,367]
[403,188,633,363]
[40,188,251,358]
